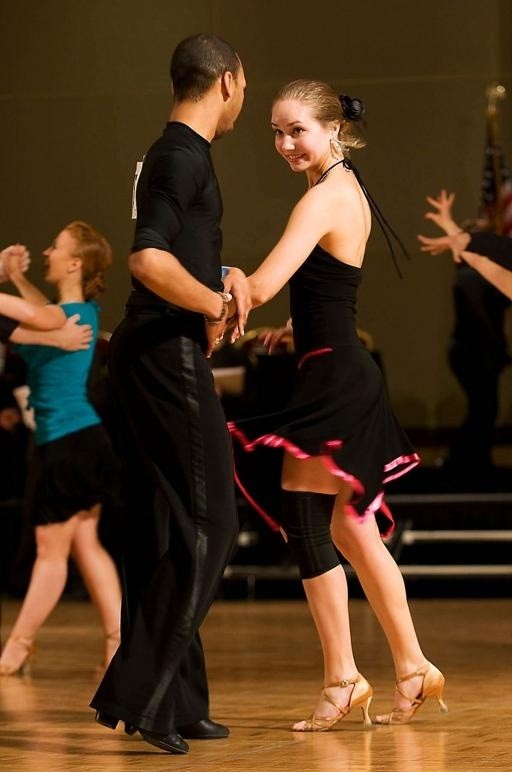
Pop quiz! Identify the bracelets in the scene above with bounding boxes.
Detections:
[204,290,226,327]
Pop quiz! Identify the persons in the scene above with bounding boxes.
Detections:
[89,30,251,756]
[227,80,447,730]
[416,172,512,471]
[2,219,118,678]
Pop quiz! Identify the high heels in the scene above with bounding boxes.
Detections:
[95,709,230,754]
[292,672,373,733]
[0,634,37,676]
[102,628,121,671]
[376,661,449,725]
